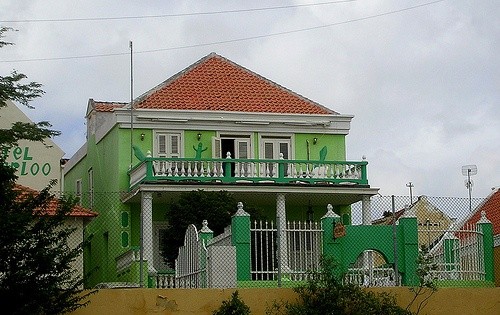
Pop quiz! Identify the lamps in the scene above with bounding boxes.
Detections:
[139,132,146,141]
[312,137,318,144]
[197,133,202,141]
